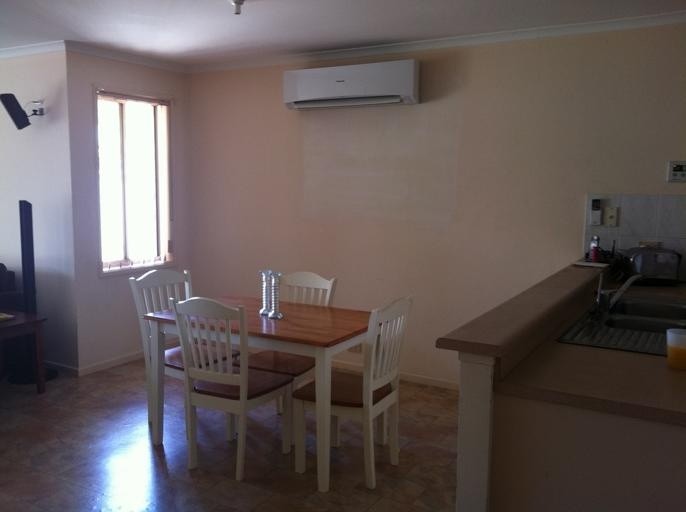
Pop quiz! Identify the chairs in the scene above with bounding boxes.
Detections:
[171,297,297,477]
[277,269,337,306]
[131,268,236,420]
[295,298,411,490]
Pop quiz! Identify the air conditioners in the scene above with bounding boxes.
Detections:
[282,58,420,110]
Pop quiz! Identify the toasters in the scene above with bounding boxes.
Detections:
[627,239,680,285]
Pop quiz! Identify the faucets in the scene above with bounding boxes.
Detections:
[594,270,644,312]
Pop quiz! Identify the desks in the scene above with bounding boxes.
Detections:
[142,293,385,494]
[1,312,48,393]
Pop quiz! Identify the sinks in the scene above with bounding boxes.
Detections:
[608,297,686,320]
[603,316,686,335]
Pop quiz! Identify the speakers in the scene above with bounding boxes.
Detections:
[19,200,37,313]
[0,93,31,130]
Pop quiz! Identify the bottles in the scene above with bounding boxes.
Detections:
[589,235,601,262]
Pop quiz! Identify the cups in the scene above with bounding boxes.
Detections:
[666,329,686,371]
